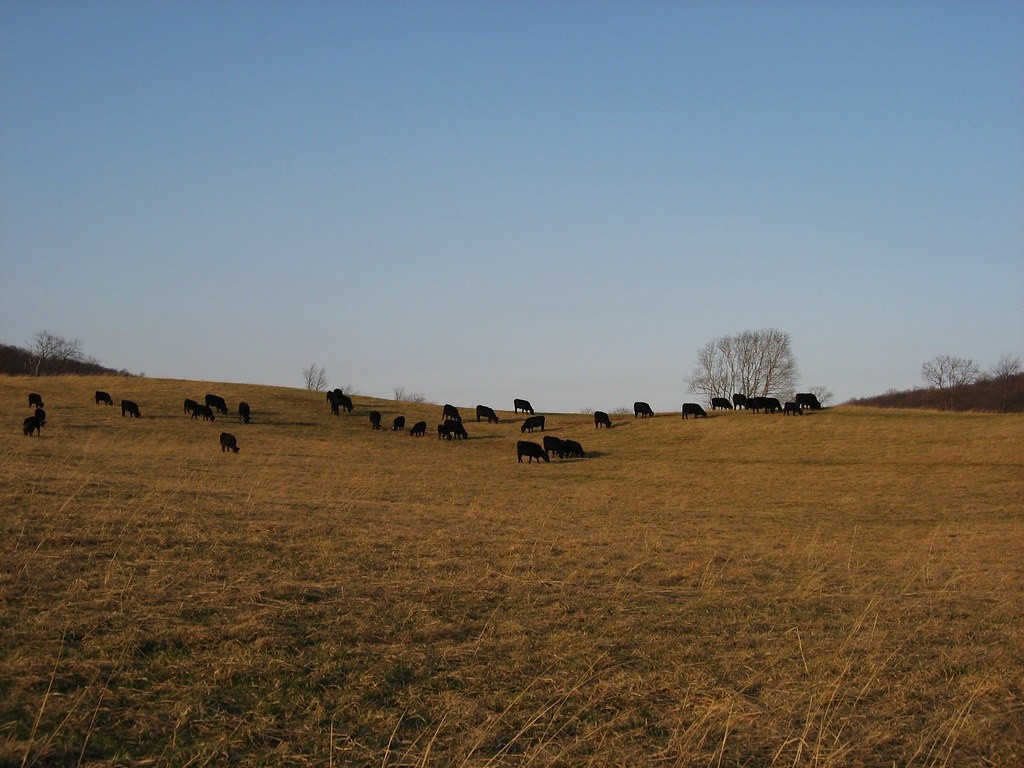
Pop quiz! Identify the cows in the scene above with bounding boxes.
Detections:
[476,404,499,424]
[595,411,613,428]
[122,400,140,418]
[184,399,215,422]
[35,408,47,427]
[712,397,733,411]
[326,388,354,415]
[29,393,44,408]
[438,404,467,441]
[205,394,229,415]
[239,402,251,424]
[96,390,113,406]
[634,402,654,418]
[22,417,40,437]
[391,416,426,437]
[514,399,584,463]
[369,410,381,430]
[220,432,240,454]
[682,403,707,419]
[733,393,821,416]
